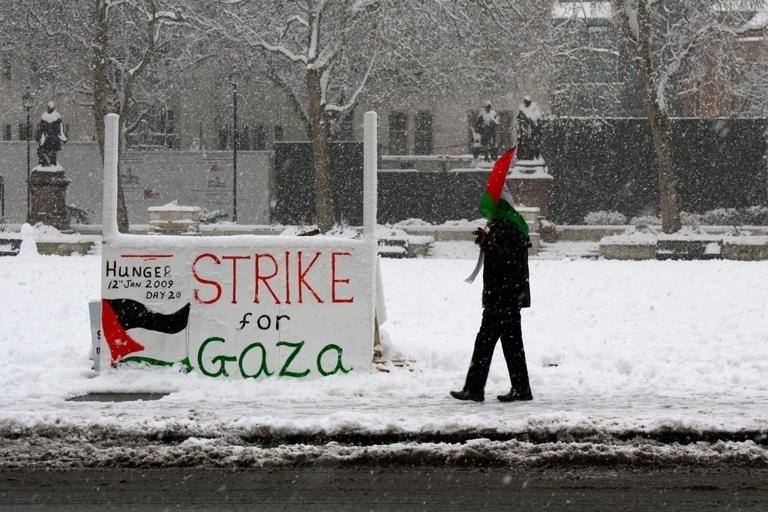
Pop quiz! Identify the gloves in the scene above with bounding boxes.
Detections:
[473,227,488,249]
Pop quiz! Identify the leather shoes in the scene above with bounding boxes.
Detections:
[450,387,485,401]
[498,388,531,403]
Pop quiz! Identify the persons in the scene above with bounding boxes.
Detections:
[449,179,535,402]
[476,100,501,160]
[35,100,66,166]
[514,95,542,161]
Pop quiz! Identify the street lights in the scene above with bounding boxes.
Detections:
[20,81,35,226]
[229,62,244,222]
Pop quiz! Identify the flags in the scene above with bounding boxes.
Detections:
[479,150,531,237]
[102,298,191,371]
